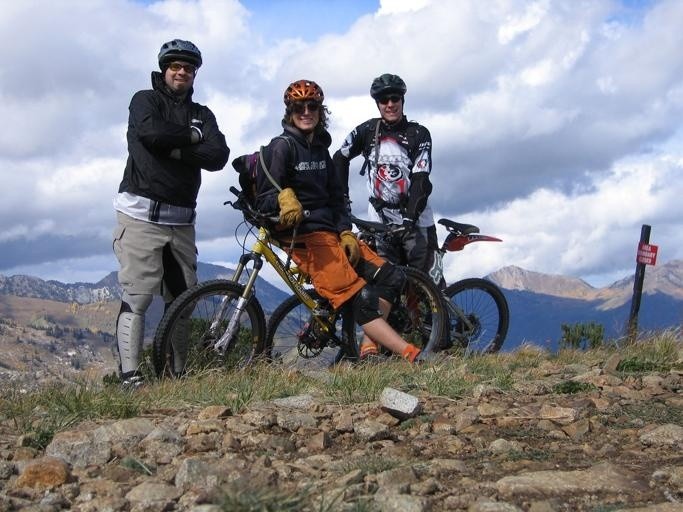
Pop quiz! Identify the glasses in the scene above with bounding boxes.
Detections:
[291,104,319,114]
[378,95,403,104]
[166,63,195,73]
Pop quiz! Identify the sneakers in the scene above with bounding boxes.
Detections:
[122,376,145,391]
[411,350,436,370]
[359,352,387,368]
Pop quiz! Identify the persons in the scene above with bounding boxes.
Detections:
[331,73,453,357]
[112,38,230,389]
[256,79,438,370]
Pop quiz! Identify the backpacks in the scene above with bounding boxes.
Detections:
[231,135,297,210]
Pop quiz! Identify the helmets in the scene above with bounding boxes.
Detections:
[284,80,324,102]
[158,39,202,67]
[370,74,407,96]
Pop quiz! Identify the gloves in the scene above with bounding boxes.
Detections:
[340,231,360,268]
[279,187,303,225]
[387,220,415,244]
[191,119,203,142]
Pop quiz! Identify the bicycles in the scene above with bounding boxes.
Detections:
[265,198,507,376]
[152,189,445,381]
